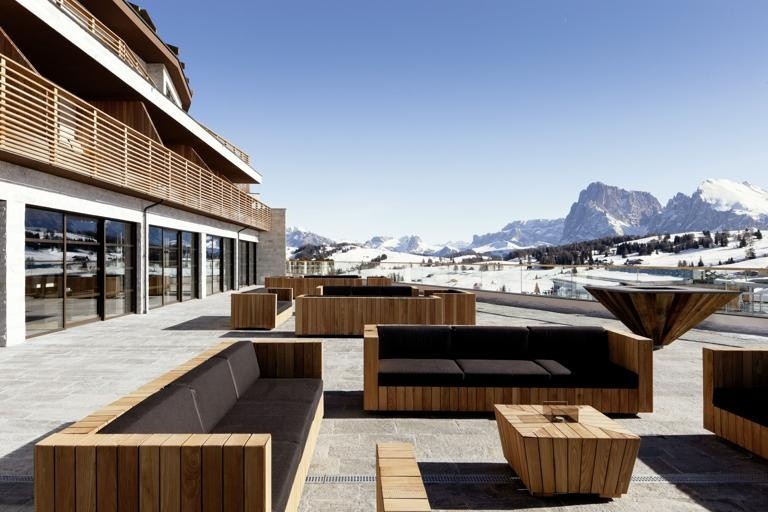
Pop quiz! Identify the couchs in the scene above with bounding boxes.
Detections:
[703,347,767,462]
[423,289,476,326]
[34,337,326,512]
[231,286,293,330]
[265,277,362,299]
[295,294,442,335]
[366,275,391,286]
[316,285,419,297]
[364,325,653,414]
